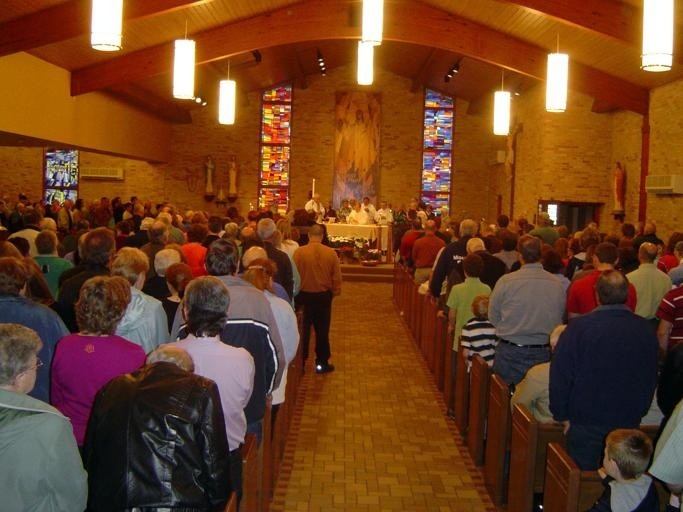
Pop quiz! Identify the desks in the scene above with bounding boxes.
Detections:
[323,223,389,263]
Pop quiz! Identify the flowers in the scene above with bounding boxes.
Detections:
[323,233,369,250]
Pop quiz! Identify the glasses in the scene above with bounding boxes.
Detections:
[15,358,45,378]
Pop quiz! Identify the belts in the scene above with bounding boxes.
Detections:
[500,339,551,350]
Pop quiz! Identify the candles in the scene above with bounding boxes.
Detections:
[311,178,315,198]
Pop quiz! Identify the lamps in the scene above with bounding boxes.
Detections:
[172,20,196,101]
[545,34,568,113]
[217,60,236,125]
[90,0,123,53]
[640,1,672,72]
[493,71,510,135]
[361,0,383,46]
[355,40,374,85]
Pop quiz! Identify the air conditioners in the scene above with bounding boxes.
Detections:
[77,165,125,182]
[488,151,505,165]
[643,173,683,195]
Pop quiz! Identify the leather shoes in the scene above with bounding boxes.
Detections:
[316,365,336,373]
